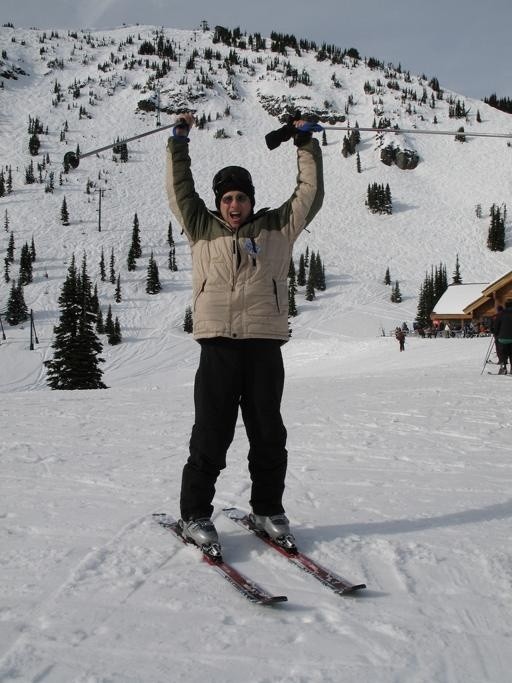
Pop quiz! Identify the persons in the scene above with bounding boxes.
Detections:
[396,298,512,374]
[163,113,325,548]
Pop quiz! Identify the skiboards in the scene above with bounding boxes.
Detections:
[150,506,365,605]
[488,371,512,374]
[486,359,512,364]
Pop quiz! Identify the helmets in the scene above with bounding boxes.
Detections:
[212,165,254,194]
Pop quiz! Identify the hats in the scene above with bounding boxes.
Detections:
[212,164,255,209]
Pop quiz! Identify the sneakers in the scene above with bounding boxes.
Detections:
[248,508,291,538]
[178,517,219,546]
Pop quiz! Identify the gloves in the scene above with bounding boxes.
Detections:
[265,110,320,150]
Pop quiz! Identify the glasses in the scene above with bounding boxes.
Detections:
[221,195,250,203]
